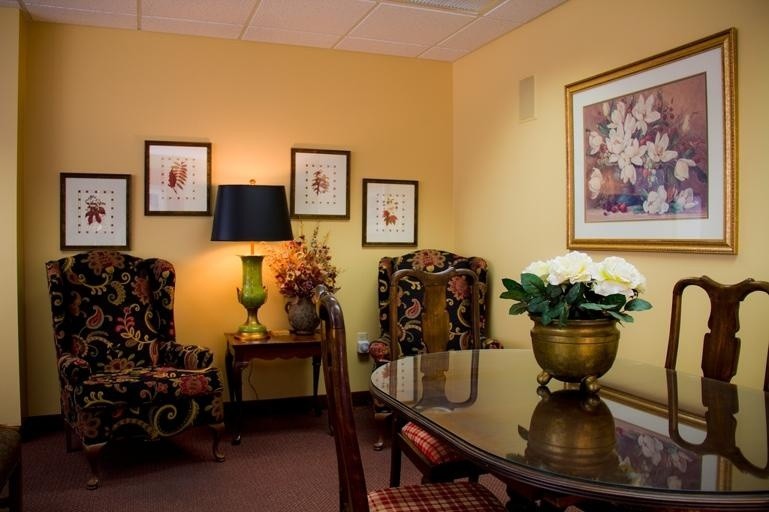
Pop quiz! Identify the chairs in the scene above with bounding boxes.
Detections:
[374,251,504,488]
[664,276,769,396]
[45,248,227,489]
[319,292,514,511]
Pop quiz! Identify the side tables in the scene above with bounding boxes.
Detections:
[222,329,327,444]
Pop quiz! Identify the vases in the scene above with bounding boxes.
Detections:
[527,314,621,390]
[284,294,321,336]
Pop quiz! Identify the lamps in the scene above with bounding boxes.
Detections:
[212,178,294,340]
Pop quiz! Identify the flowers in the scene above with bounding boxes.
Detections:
[261,214,342,299]
[498,250,651,331]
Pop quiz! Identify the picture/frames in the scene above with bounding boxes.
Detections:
[145,142,211,216]
[564,27,739,255]
[362,178,419,246]
[290,148,350,220]
[59,173,132,250]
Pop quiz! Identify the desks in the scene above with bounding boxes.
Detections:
[369,345,769,512]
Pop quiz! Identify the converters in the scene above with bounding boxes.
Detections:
[358,341,369,353]
